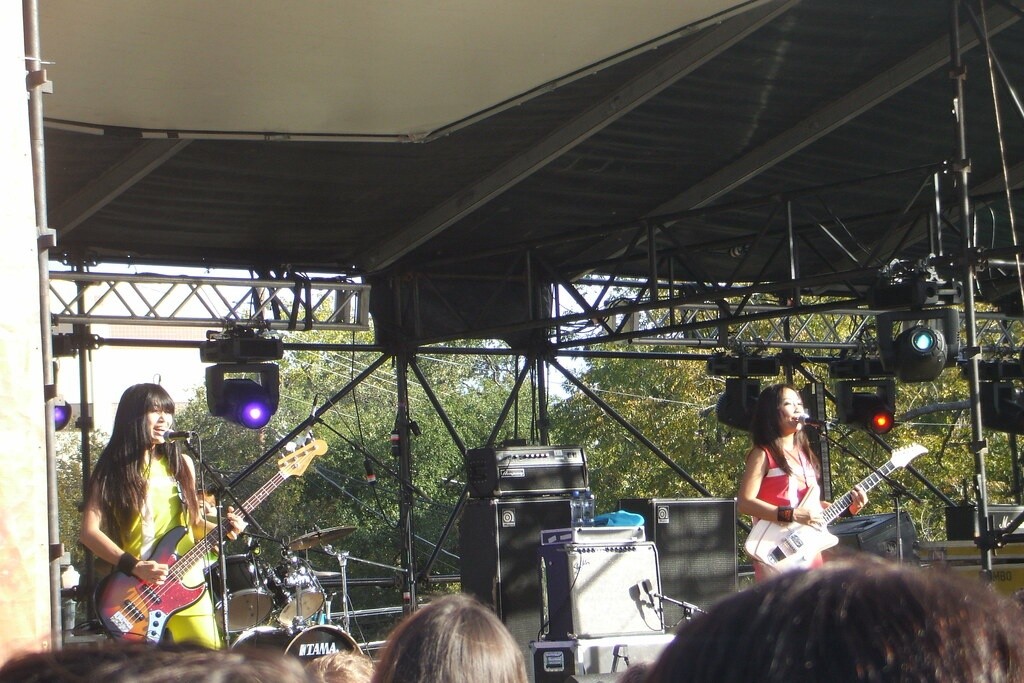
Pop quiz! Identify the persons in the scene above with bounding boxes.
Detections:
[79,384,248,649]
[739,383,869,586]
[616,555,1023,682]
[0,592,527,683]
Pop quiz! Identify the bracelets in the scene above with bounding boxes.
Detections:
[118,551,140,577]
[778,505,794,523]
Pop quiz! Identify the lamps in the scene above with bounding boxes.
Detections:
[875,306,961,385]
[977,377,1023,435]
[53,402,72,430]
[716,377,763,430]
[208,363,282,429]
[837,379,896,433]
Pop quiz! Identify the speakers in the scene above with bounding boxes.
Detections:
[618,496,739,632]
[821,510,919,568]
[542,542,665,639]
[459,494,594,683]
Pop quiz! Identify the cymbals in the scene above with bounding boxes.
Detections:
[312,569,341,578]
[287,525,358,552]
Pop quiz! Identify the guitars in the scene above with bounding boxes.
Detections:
[92,428,329,646]
[743,441,929,575]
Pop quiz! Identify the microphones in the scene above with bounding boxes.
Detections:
[799,414,837,428]
[641,578,657,611]
[164,429,197,443]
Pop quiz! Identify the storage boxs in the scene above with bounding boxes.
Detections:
[822,510,918,566]
[945,503,1024,542]
[532,635,675,683]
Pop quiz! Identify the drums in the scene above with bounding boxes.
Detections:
[203,553,272,634]
[267,557,326,627]
[231,625,365,668]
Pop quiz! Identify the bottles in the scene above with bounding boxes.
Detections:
[583,491,595,528]
[570,490,586,527]
